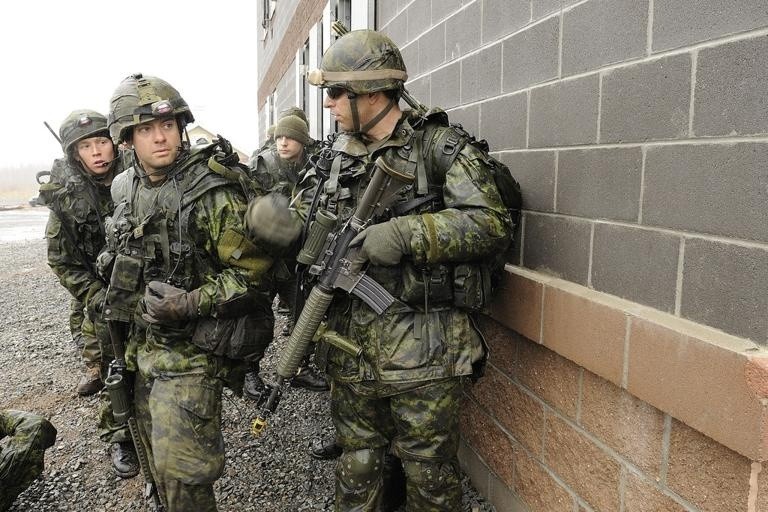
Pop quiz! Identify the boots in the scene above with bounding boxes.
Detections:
[290,355,330,392]
[76,359,104,396]
[312,432,343,460]
[243,364,266,401]
[280,312,296,336]
[109,442,140,479]
[382,444,404,476]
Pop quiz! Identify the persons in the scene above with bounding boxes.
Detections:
[247,30,516,512]
[247,107,343,460]
[0,409,58,512]
[96,73,275,512]
[46,109,140,479]
[70,296,104,396]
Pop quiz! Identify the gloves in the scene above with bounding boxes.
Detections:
[141,282,203,328]
[251,193,300,247]
[348,216,414,270]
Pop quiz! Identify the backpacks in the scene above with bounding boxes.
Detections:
[38,150,131,226]
[431,119,522,318]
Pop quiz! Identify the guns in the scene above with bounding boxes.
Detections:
[97,300,165,512]
[253,156,416,431]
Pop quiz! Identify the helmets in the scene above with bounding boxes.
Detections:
[59,109,108,165]
[277,105,309,132]
[306,29,408,100]
[274,115,310,145]
[107,72,196,146]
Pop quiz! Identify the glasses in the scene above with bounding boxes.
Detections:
[324,87,350,100]
